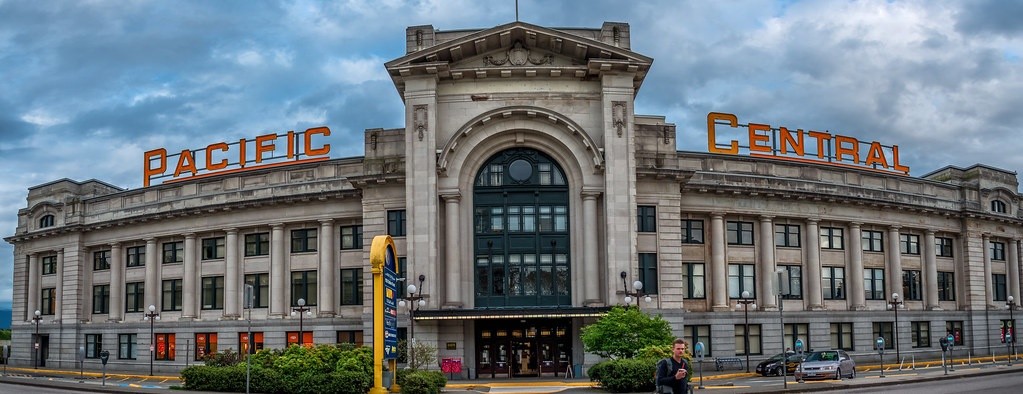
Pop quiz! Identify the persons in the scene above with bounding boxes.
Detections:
[654,339,690,394]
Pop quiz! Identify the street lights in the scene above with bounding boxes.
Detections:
[399,285,426,371]
[291,298,312,349]
[888,293,904,365]
[31,309,43,370]
[624,281,652,314]
[145,304,161,375]
[735,290,757,374]
[1005,296,1017,356]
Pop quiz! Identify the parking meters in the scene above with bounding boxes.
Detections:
[1006,332,1013,368]
[694,342,705,390]
[939,337,949,375]
[875,337,886,378]
[947,334,955,372]
[79,347,84,383]
[794,339,805,383]
[2,344,8,376]
[99,351,110,387]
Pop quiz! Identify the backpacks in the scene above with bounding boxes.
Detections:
[655,358,674,393]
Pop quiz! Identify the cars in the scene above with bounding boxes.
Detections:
[756,351,814,377]
[795,350,857,380]
